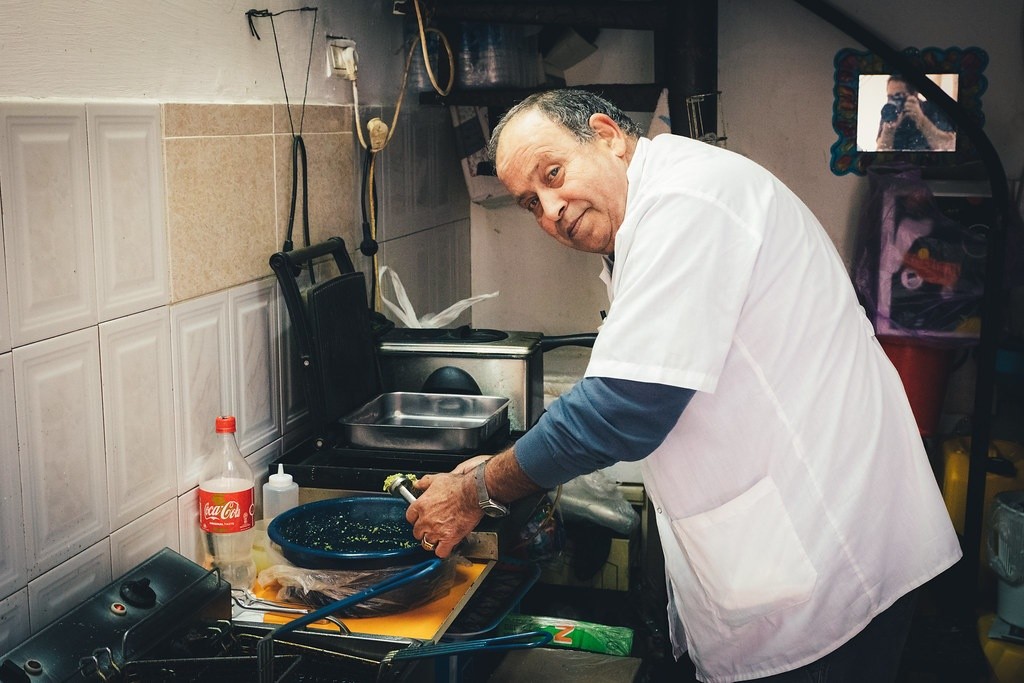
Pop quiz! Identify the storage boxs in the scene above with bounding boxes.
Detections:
[496,583,637,657]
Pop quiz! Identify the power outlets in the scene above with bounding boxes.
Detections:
[325,36,360,80]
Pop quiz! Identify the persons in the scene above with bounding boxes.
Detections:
[406,90,1002,683]
[877,76,956,151]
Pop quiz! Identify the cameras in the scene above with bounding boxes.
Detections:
[881,92,906,123]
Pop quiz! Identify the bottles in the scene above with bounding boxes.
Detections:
[197,418,257,598]
[262,464,298,529]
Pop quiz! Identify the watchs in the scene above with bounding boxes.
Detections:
[475,464,510,517]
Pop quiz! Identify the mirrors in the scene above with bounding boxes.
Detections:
[830,46,989,177]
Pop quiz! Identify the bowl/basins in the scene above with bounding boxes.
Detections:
[267,496,460,618]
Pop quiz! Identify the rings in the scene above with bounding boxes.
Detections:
[422,535,433,552]
